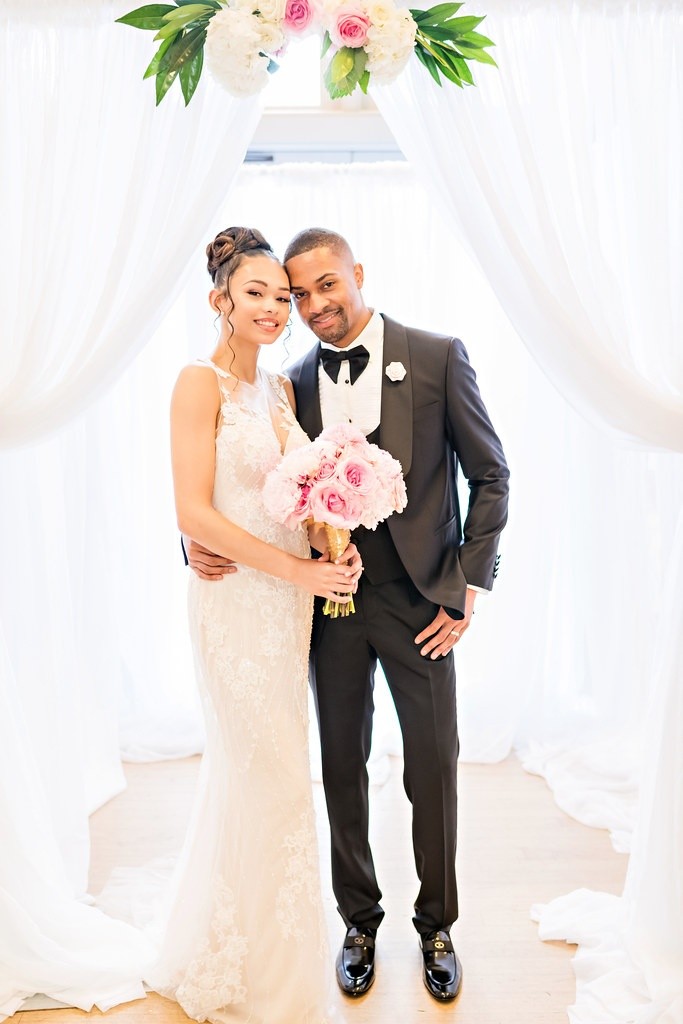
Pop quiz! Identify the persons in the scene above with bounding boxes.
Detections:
[20,225,362,1024]
[180,229,508,1005]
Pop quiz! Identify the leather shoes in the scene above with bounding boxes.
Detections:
[335,927,377,996]
[418,928,462,1001]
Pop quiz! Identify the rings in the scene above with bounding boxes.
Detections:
[451,631,459,636]
[361,567,364,570]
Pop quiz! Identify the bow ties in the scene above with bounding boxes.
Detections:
[319,345,370,385]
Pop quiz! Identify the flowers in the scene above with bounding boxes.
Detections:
[115,0,499,108]
[264,425,409,618]
[385,362,407,382]
